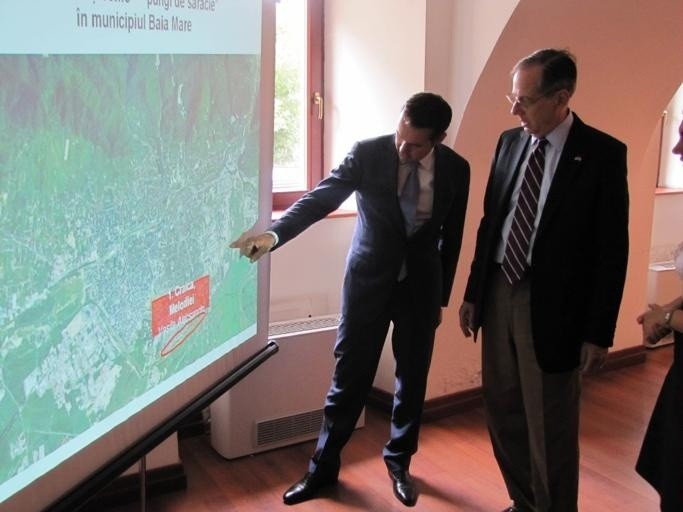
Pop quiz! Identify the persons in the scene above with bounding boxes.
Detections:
[458,49,628,511]
[635,113,683,512]
[244,92,470,506]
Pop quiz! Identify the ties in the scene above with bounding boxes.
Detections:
[398,163,421,281]
[501,138,550,284]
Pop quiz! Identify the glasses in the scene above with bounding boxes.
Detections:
[505,88,560,106]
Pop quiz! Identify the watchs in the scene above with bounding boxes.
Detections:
[665,307,679,330]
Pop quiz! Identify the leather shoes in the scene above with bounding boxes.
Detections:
[388,468,417,507]
[283,473,338,503]
